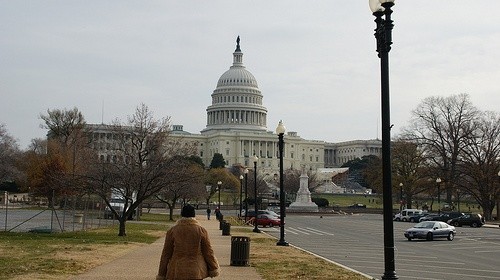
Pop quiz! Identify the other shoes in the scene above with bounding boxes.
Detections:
[208,218,209,220]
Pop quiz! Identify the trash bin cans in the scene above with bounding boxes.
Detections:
[230,236,250,266]
[216,213,220,219]
[222,223,230,236]
[220,220,225,230]
[220,215,223,221]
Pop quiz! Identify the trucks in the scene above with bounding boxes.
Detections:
[105,188,137,219]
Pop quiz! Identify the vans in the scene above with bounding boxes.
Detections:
[395,209,422,221]
[250,210,280,218]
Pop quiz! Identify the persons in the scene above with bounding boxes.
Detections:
[156,204,221,280]
[400,200,405,210]
[206,206,211,220]
[214,206,219,219]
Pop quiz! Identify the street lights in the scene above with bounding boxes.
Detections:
[218,181,222,211]
[240,174,244,217]
[276,120,290,246]
[252,154,260,233]
[244,169,249,223]
[370,0,401,280]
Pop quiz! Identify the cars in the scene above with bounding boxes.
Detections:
[448,213,486,227]
[403,221,456,241]
[408,213,428,221]
[419,214,450,222]
[250,214,286,227]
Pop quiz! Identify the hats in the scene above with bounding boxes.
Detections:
[181,204,195,217]
